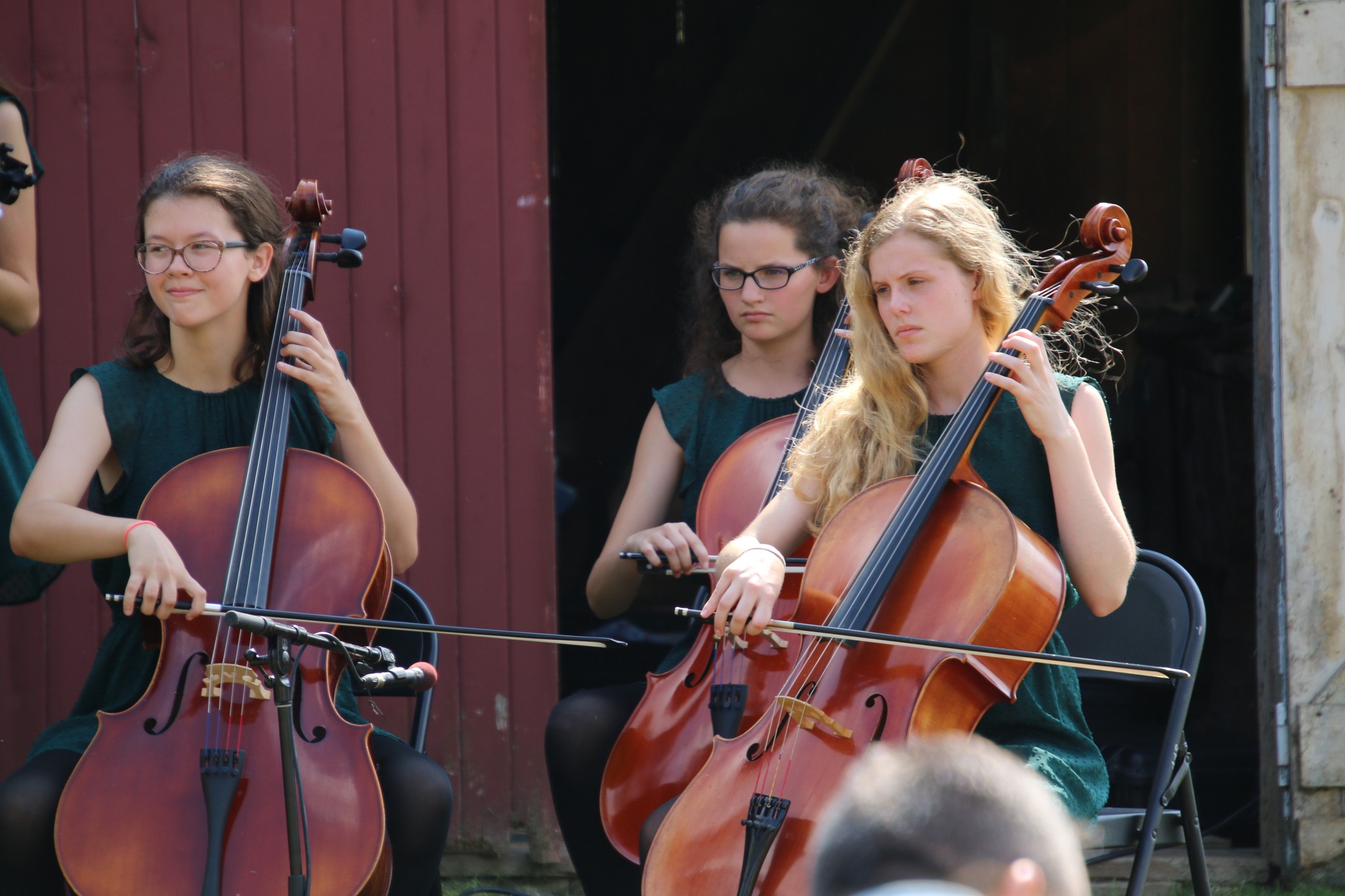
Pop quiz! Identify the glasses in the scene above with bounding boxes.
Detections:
[133,241,248,275]
[708,253,831,291]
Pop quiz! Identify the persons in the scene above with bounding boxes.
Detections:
[1,87,66,606]
[0,155,453,896]
[811,730,1092,896]
[700,174,1139,828]
[545,166,867,896]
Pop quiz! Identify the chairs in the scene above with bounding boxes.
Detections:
[351,577,437,752]
[1057,550,1212,896]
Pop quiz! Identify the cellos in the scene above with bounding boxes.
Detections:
[639,201,1153,896]
[52,178,393,896]
[601,154,941,864]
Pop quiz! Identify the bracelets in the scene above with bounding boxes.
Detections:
[123,521,158,552]
[743,548,786,568]
